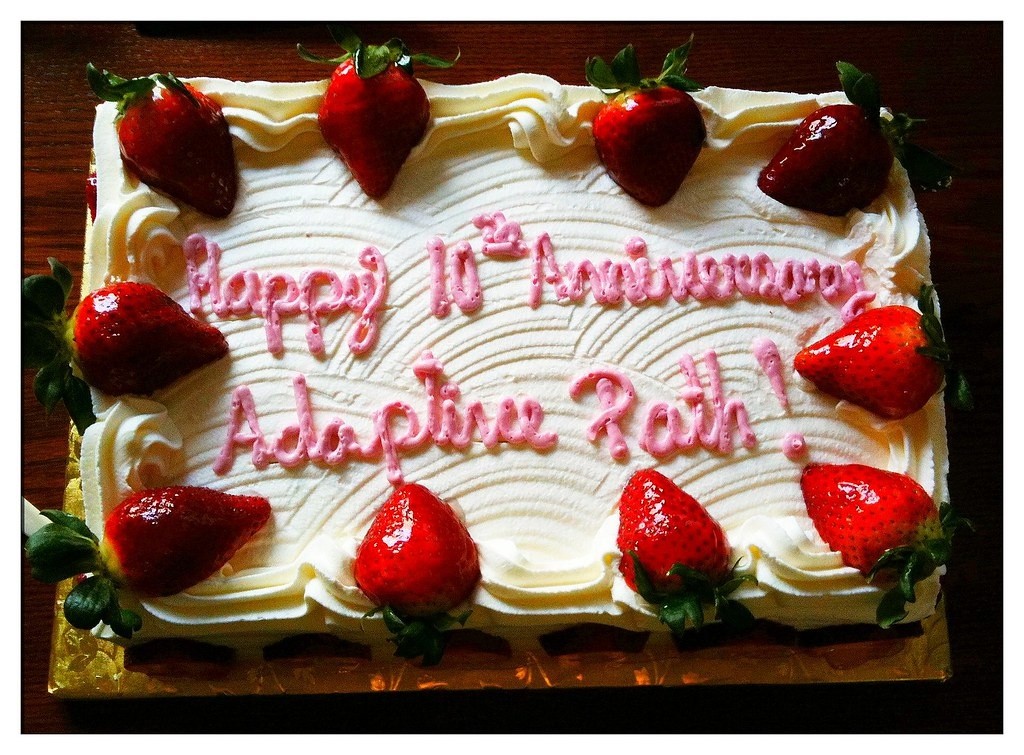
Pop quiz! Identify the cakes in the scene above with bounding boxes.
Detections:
[24,36,987,663]
